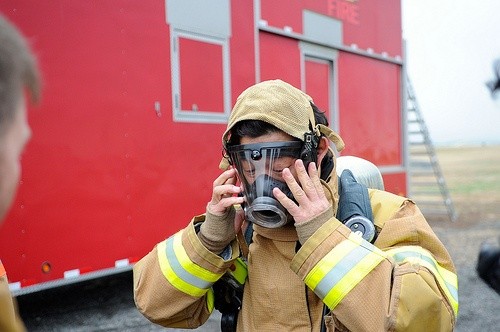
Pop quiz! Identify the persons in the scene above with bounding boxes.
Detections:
[130,75,459,331]
[0,16,46,331]
